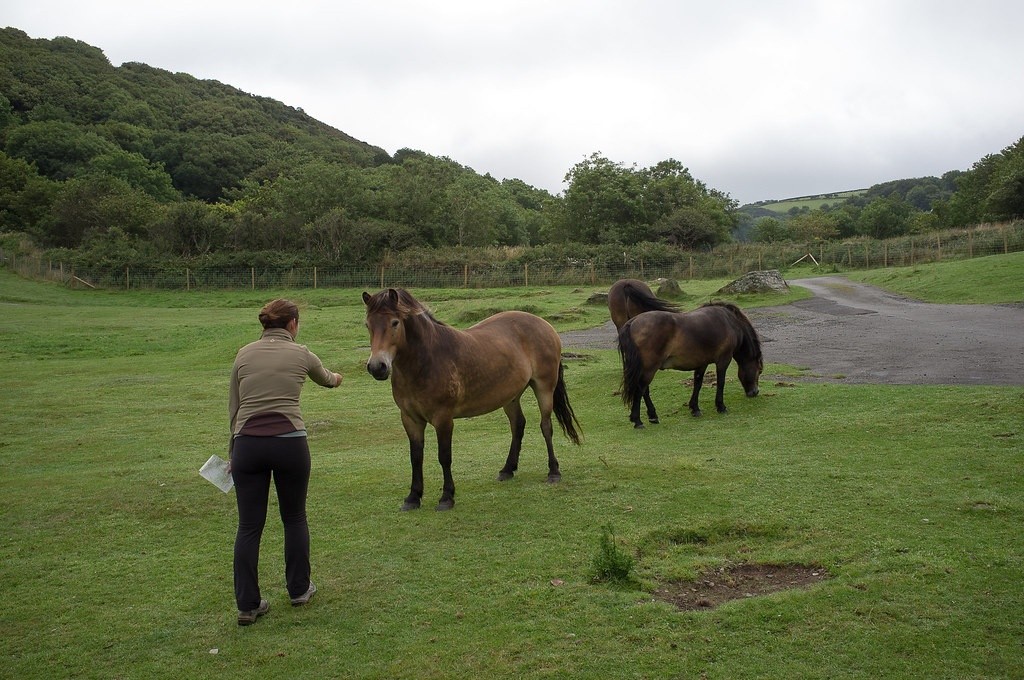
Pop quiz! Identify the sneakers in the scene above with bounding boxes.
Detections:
[238,600,269,625]
[291,580,317,607]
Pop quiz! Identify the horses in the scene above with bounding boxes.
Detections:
[361,286,586,512]
[607,278,686,397]
[617,298,763,429]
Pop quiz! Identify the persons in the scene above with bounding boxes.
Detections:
[230,300,342,627]
